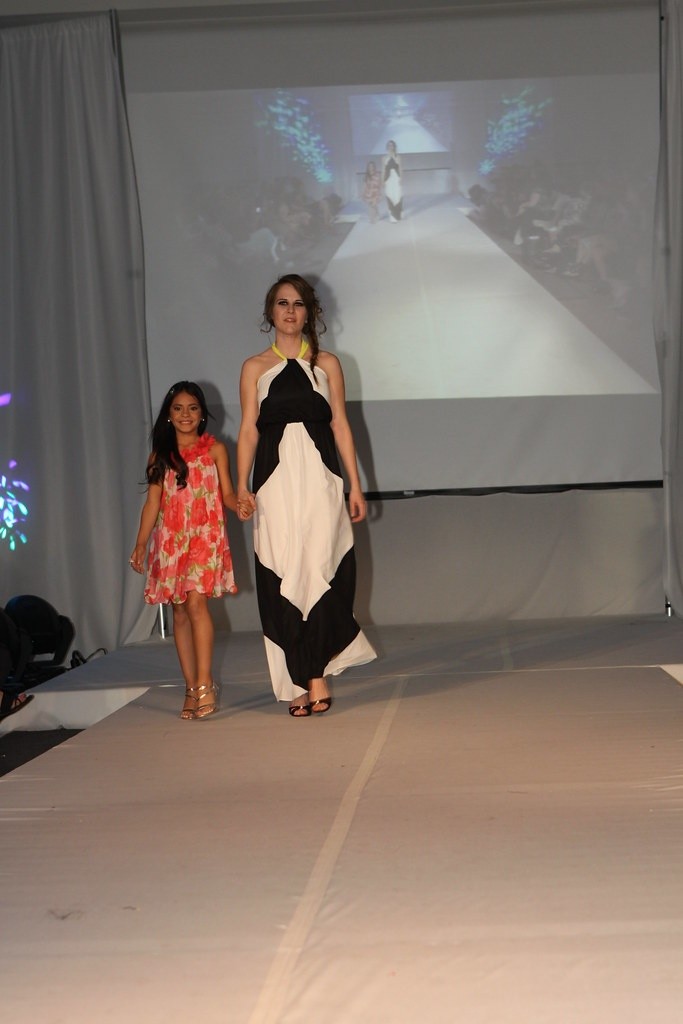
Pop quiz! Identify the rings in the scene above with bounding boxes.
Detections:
[129,559,135,562]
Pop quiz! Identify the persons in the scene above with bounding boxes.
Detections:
[466,165,642,317]
[381,140,403,223]
[127,380,253,717]
[237,273,368,717]
[361,162,382,223]
[238,177,346,282]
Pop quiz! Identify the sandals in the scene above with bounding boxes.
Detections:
[0,692,34,723]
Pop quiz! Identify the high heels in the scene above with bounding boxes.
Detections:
[180,687,197,719]
[309,697,332,713]
[289,705,309,716]
[197,680,220,718]
